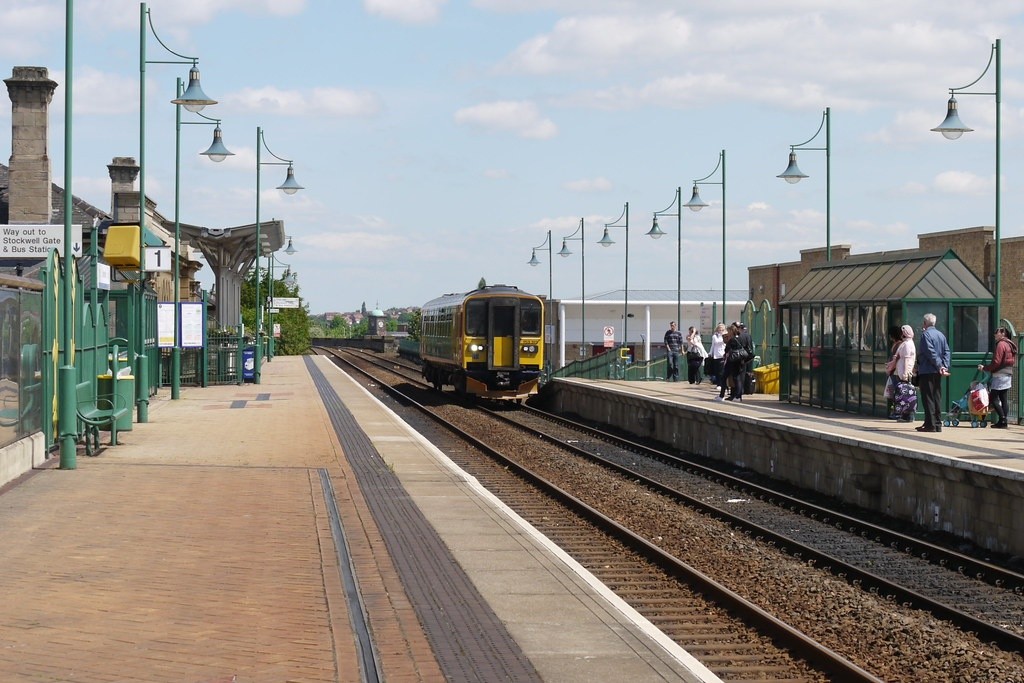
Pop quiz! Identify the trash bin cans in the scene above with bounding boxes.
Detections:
[243,349,255,383]
[96,375,135,431]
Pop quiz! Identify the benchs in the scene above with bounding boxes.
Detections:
[0,382,40,435]
[75,381,128,456]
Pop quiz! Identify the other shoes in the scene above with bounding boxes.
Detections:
[725,397,733,402]
[696,378,702,385]
[991,418,1007,429]
[690,380,695,384]
[674,379,678,382]
[665,378,670,382]
[713,395,725,402]
[732,397,741,403]
[897,418,914,422]
[936,425,942,432]
[915,423,936,432]
[716,385,727,393]
[887,414,900,420]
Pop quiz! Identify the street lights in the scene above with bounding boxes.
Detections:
[597,201,628,347]
[254,127,305,383]
[267,218,299,363]
[172,76,235,402]
[931,38,1001,330]
[775,108,830,260]
[138,3,218,424]
[526,230,553,371]
[557,218,586,361]
[682,150,727,325]
[644,213,681,333]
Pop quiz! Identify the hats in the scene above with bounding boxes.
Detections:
[901,325,914,338]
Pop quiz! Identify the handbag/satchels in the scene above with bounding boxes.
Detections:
[885,355,898,376]
[968,391,989,415]
[743,372,756,395]
[704,355,715,376]
[743,345,755,363]
[884,376,895,400]
[911,371,920,387]
[690,346,701,362]
[893,380,918,415]
[970,383,990,412]
[729,337,748,362]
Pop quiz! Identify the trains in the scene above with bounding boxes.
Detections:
[420,283,547,406]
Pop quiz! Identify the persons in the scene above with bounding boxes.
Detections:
[917,313,951,432]
[978,327,1019,428]
[681,321,755,402]
[884,325,931,428]
[664,321,685,382]
[948,380,979,414]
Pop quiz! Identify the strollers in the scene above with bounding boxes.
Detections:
[943,369,991,428]
